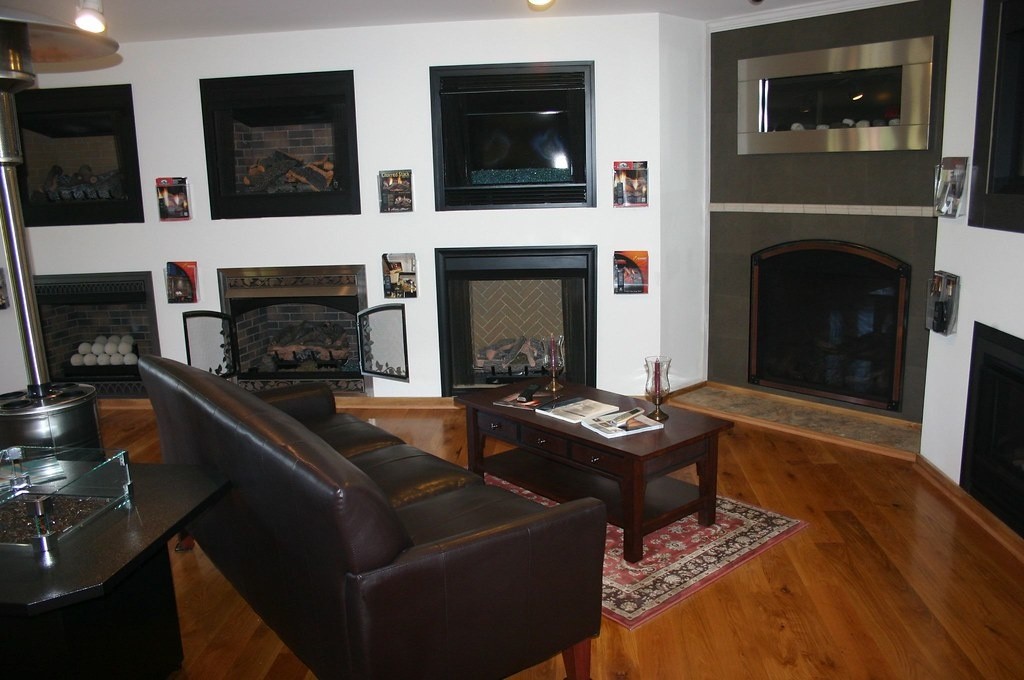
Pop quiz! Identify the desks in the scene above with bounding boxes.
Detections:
[1,459,223,680]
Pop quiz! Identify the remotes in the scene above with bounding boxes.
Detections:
[517,384,541,402]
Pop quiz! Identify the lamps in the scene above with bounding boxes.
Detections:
[74,1,107,34]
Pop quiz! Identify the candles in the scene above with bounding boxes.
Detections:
[550,332,557,368]
[654,356,661,397]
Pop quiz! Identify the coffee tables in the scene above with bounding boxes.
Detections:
[453,375,735,564]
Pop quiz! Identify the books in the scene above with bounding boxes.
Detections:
[580,410,665,440]
[491,385,565,411]
[536,394,620,424]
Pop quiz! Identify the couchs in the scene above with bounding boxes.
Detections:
[139,353,607,680]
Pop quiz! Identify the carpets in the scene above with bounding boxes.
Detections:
[463,464,810,629]
